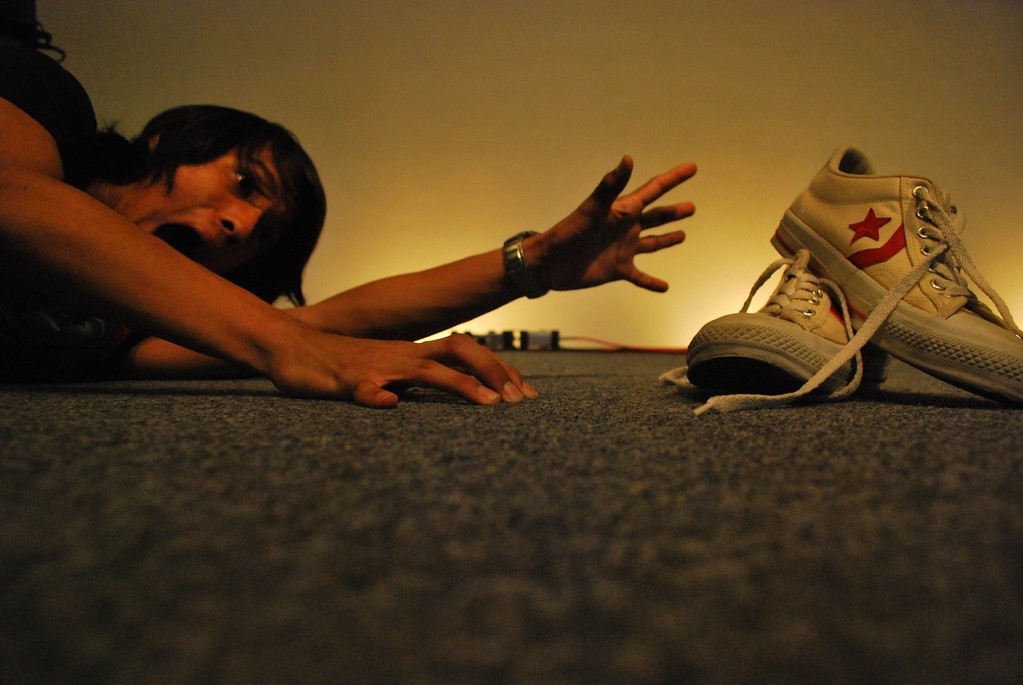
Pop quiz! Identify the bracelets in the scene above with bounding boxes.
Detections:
[502,230,550,300]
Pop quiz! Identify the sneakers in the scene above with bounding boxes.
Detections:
[658,249,889,401]
[691,144,1023,415]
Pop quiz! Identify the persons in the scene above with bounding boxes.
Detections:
[0,35,697,406]
